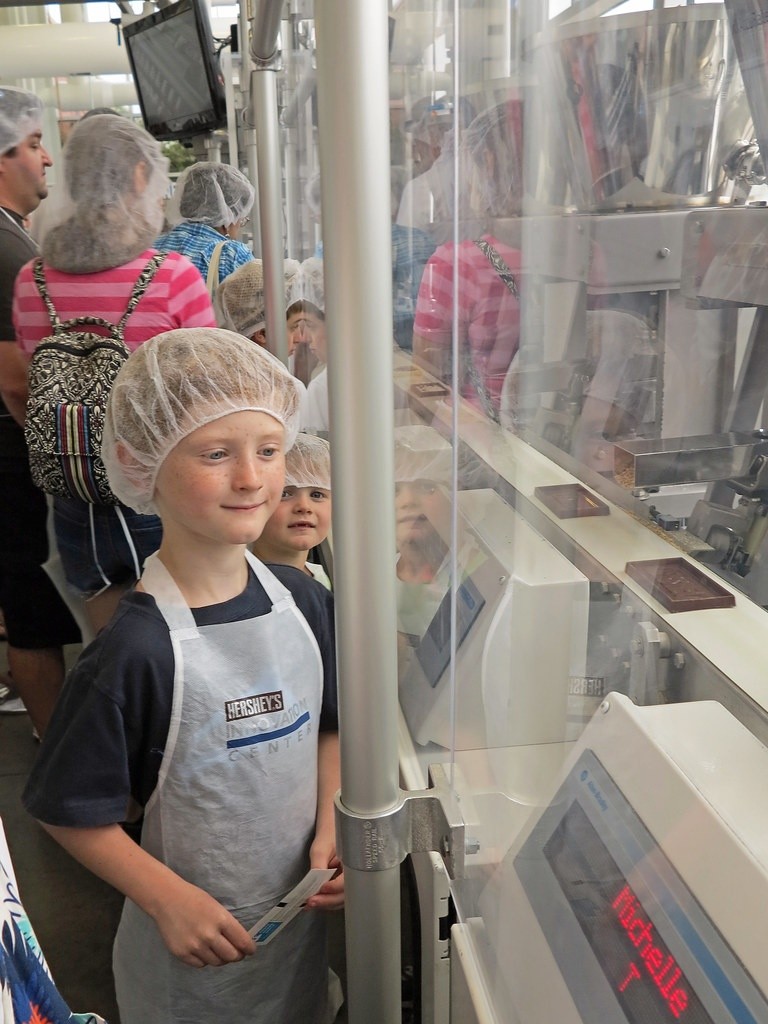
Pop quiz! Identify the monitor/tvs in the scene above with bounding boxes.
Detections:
[122,0,228,141]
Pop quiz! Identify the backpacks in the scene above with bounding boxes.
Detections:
[25,249,168,504]
[464,237,588,454]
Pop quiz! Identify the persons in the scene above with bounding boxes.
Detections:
[302,256,328,432]
[0,87,328,749]
[22,325,342,1023]
[395,71,684,820]
[255,432,335,605]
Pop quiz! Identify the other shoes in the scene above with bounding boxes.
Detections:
[0,682,27,712]
